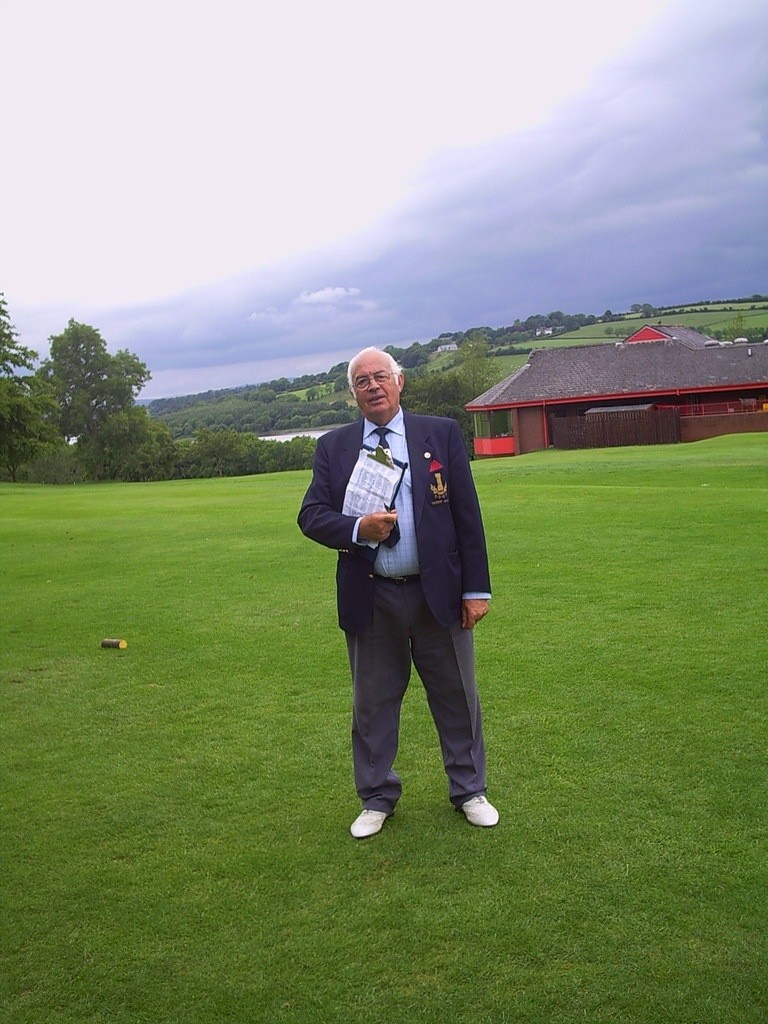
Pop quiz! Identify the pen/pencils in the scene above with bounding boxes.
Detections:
[383,502,399,532]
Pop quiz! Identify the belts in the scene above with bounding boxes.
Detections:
[372,574,420,587]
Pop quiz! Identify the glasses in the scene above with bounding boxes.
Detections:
[349,371,398,391]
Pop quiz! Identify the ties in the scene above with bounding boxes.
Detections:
[372,427,401,549]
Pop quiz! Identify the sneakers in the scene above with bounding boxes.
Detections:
[455,795,499,826]
[350,809,395,839]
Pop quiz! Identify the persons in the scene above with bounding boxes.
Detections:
[296,346,501,838]
